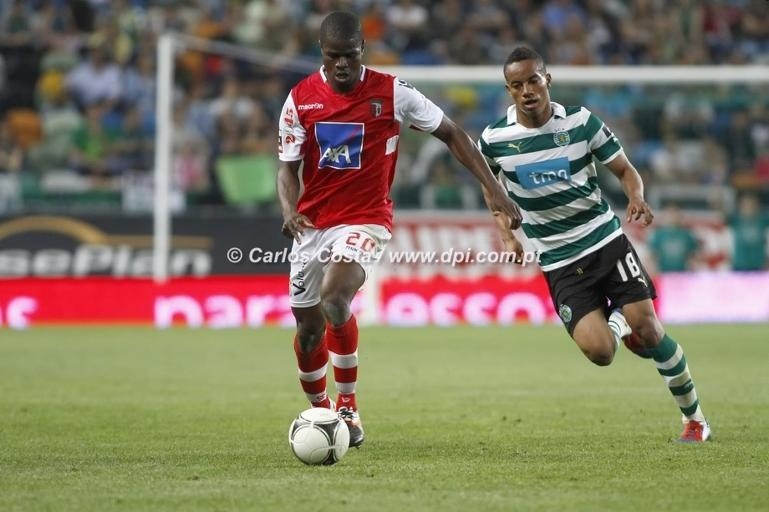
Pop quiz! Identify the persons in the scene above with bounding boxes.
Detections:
[472,46,713,446]
[273,11,525,451]
[0,0,768,275]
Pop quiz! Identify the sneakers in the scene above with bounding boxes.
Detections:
[337,406,364,448]
[613,308,652,358]
[676,421,710,442]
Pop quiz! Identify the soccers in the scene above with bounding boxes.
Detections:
[288,408,350,465]
